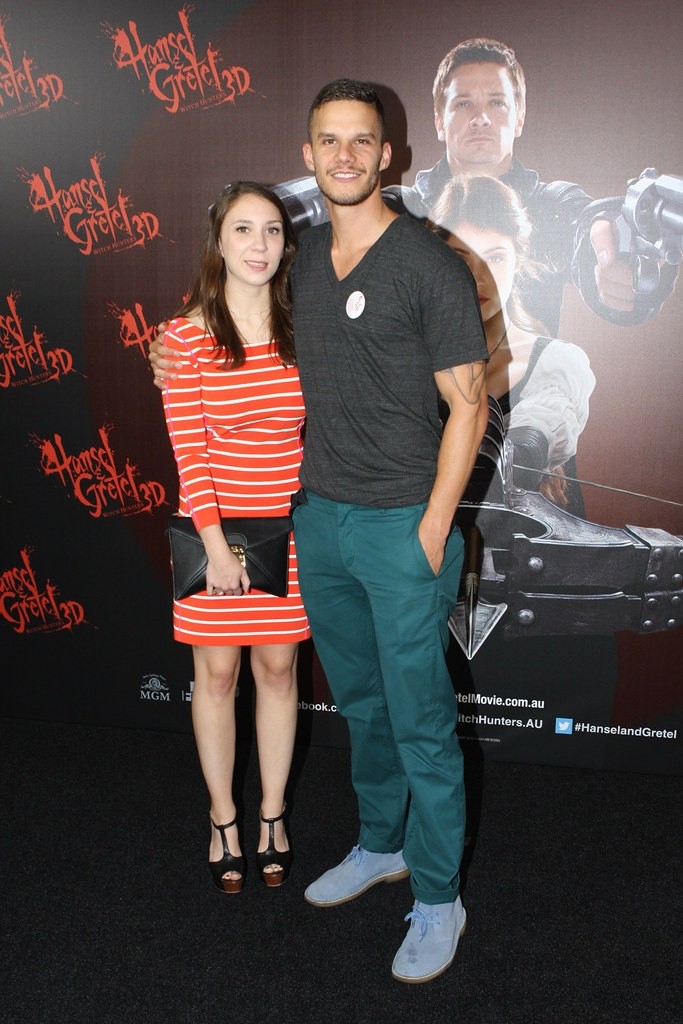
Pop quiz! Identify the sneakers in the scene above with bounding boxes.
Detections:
[391,893,467,985]
[303,844,410,908]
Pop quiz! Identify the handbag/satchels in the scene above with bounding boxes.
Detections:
[163,516,293,602]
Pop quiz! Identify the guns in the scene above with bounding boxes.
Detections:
[207,172,332,242]
[598,166,683,295]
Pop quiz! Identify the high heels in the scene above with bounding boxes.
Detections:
[208,806,245,894]
[256,803,291,887]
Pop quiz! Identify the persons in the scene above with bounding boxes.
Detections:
[382,37,678,734]
[149,79,490,985]
